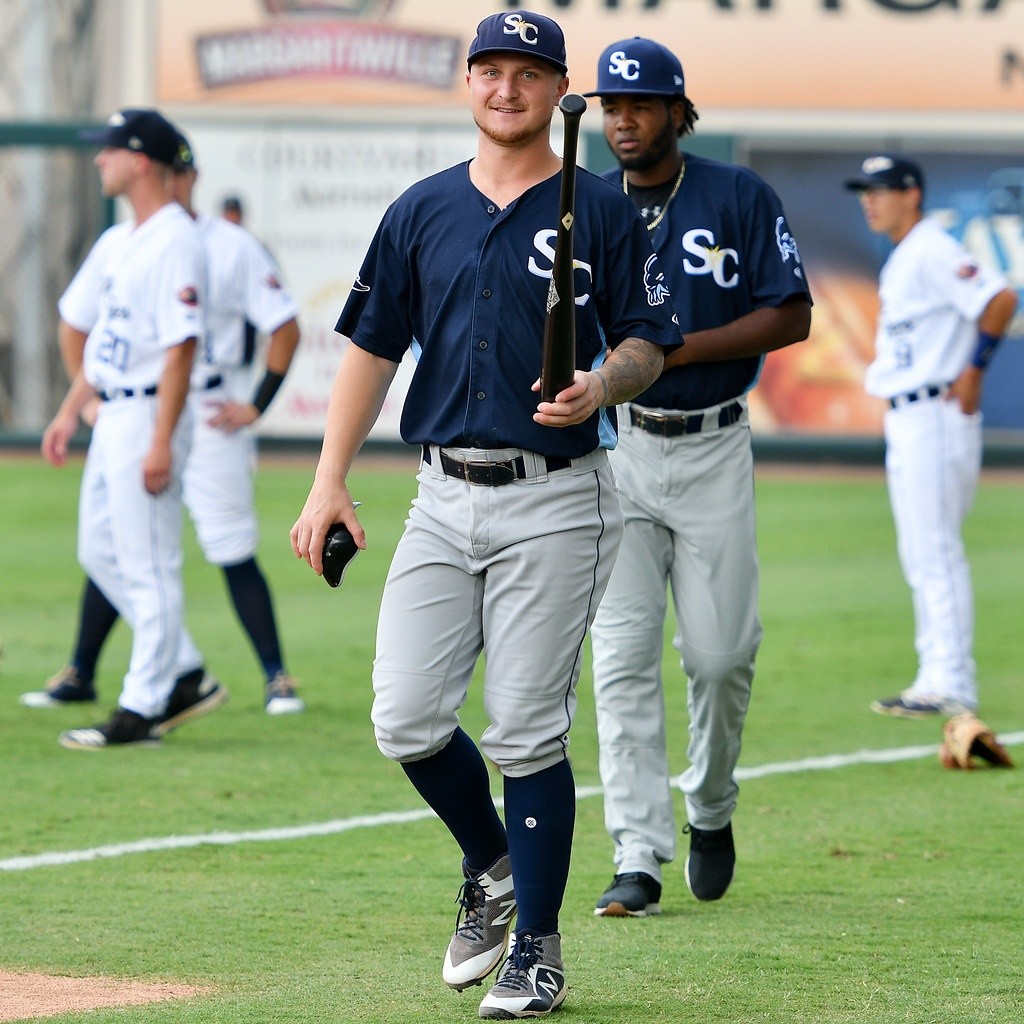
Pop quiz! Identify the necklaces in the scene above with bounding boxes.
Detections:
[622,161,685,230]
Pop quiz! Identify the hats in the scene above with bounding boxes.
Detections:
[81,106,177,164]
[466,11,568,78]
[166,132,194,171]
[843,156,923,195]
[582,36,684,99]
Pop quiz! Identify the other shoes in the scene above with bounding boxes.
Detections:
[874,696,944,717]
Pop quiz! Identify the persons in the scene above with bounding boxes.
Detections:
[288,10,686,1021]
[20,125,306,714]
[584,35,815,916]
[224,198,241,223]
[38,108,225,750]
[841,153,1020,720]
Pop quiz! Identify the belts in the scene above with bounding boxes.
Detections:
[96,386,156,402]
[209,374,223,388]
[423,446,570,486]
[629,403,743,436]
[888,382,955,407]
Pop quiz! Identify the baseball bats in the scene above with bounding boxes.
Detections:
[540,93,588,405]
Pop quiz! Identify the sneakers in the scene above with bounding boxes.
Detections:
[441,849,518,991]
[682,814,737,902]
[480,931,568,1018]
[151,668,230,741]
[59,701,154,749]
[265,674,302,713]
[595,866,661,916]
[20,672,94,707]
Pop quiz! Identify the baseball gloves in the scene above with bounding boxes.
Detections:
[939,709,1015,771]
